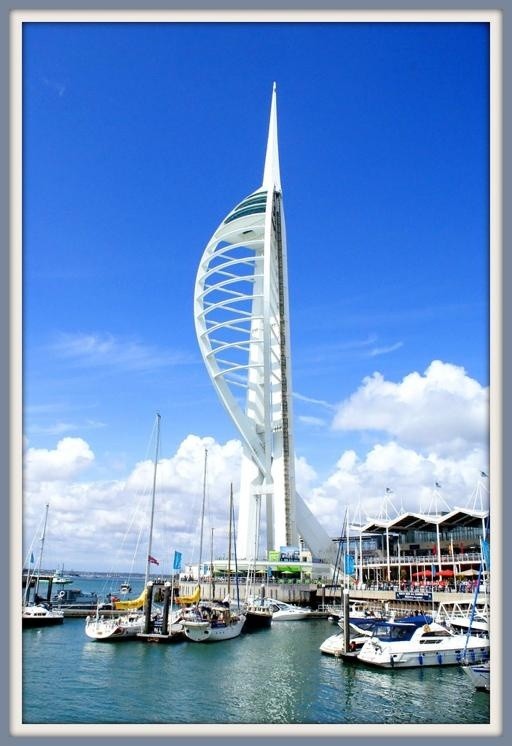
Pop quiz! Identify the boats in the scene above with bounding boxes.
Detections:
[32,563,144,623]
[37,570,74,584]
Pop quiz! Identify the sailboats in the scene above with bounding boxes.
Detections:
[82,407,314,647]
[22,501,68,628]
[319,508,490,693]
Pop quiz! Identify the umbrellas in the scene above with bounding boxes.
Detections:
[434,569,454,586]
[409,570,432,583]
[455,569,482,585]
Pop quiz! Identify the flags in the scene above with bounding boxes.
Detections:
[345,554,356,576]
[482,542,492,575]
[481,472,486,478]
[384,487,392,494]
[173,550,184,572]
[148,555,162,568]
[435,482,441,488]
